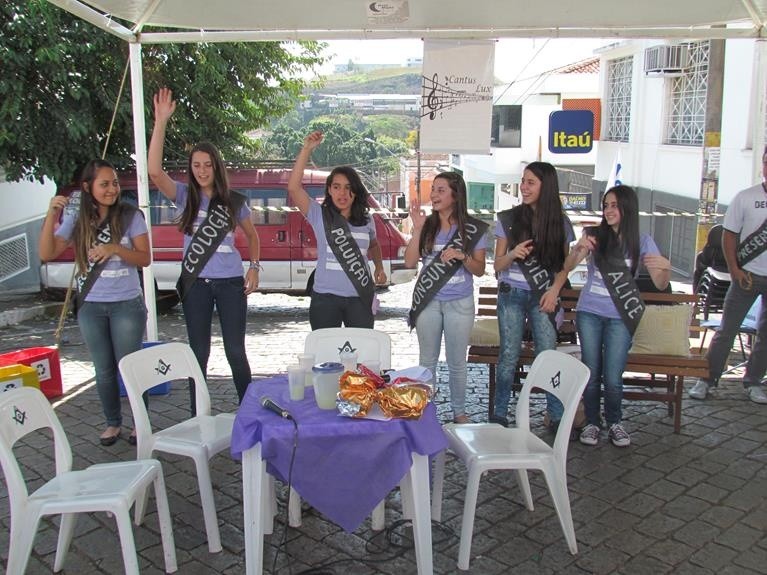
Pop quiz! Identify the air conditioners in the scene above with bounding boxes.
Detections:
[644,44,689,76]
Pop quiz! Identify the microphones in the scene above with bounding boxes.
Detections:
[259,396,289,419]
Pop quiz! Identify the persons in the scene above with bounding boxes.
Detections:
[493,161,565,433]
[39,158,151,445]
[404,172,491,422]
[288,131,386,332]
[148,89,259,417]
[689,147,767,403]
[564,185,670,446]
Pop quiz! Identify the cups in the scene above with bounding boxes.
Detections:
[287,365,305,401]
[365,360,381,376]
[298,353,316,387]
[341,352,358,371]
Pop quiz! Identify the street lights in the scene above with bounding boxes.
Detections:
[362,137,421,209]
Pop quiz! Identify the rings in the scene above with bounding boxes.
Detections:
[585,243,588,246]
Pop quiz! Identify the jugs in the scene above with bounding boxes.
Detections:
[311,362,344,410]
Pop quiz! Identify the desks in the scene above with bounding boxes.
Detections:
[231,377,448,575]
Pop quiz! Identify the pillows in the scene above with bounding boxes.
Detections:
[469,319,501,346]
[627,304,694,356]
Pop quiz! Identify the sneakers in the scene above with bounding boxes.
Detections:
[608,424,631,446]
[689,379,710,399]
[579,423,600,445]
[742,385,767,403]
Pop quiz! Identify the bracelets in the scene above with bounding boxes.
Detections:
[249,260,264,271]
[463,253,472,263]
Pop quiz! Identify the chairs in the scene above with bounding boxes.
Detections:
[304,327,392,371]
[0,342,233,575]
[431,349,592,571]
[699,296,761,362]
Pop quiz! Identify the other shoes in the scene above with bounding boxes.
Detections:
[549,418,577,440]
[99,427,122,446]
[128,435,137,445]
[493,415,507,428]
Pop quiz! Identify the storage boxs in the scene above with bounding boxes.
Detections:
[0,348,63,399]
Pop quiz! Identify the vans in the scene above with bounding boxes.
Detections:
[38,159,422,313]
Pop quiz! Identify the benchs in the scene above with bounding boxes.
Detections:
[468,287,710,435]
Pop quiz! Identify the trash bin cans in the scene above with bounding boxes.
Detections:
[0,347,63,399]
[0,364,38,394]
[117,342,169,396]
